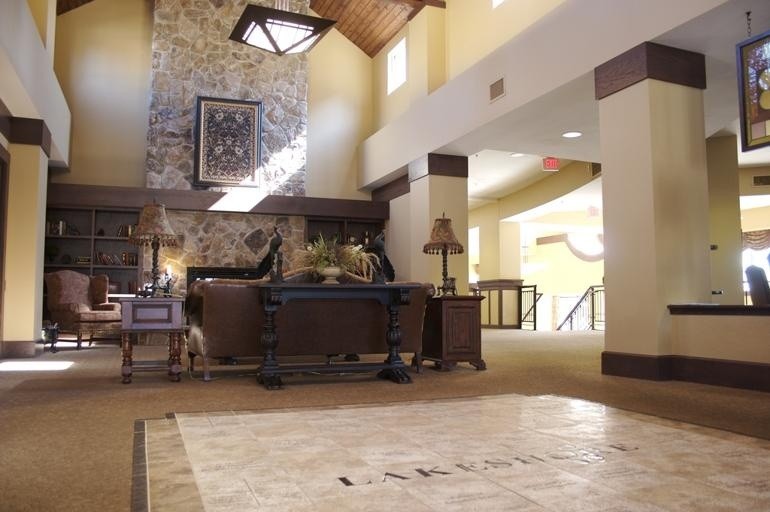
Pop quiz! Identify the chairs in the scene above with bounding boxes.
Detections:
[44,270,123,353]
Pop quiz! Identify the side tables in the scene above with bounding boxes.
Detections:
[119,298,186,383]
[412,296,487,371]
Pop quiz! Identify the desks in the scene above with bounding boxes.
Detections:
[248,282,421,389]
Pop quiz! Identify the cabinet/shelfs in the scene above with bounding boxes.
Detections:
[305,216,383,246]
[44,204,139,278]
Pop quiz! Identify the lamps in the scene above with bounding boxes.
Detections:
[129,198,178,298]
[229,0,337,56]
[423,212,463,296]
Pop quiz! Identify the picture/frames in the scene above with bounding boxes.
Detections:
[736,31,770,152]
[194,95,263,188]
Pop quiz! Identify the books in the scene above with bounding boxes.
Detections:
[117,224,139,239]
[46,219,68,237]
[309,228,374,250]
[128,279,137,294]
[72,250,137,267]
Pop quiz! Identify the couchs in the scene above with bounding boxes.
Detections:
[187,268,435,381]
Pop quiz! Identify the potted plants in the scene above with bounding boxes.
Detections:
[289,233,381,285]
